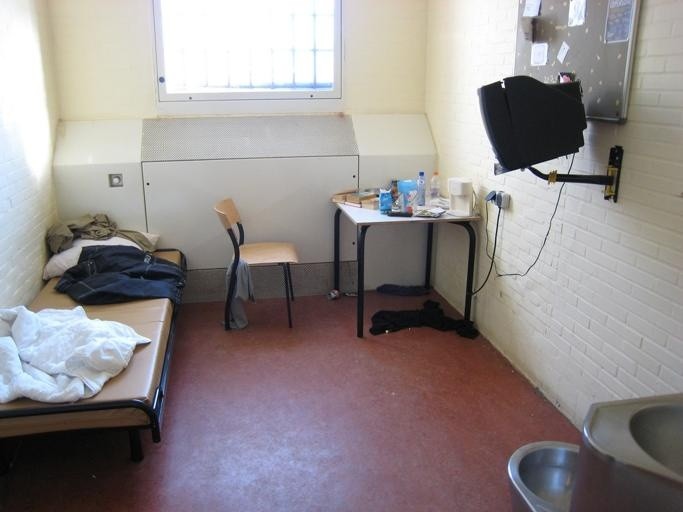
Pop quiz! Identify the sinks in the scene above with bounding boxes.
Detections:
[507,441,580,510]
[629,407,683,477]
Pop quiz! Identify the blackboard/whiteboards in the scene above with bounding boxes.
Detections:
[513,0,642,125]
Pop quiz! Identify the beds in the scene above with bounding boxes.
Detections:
[0,249,185,463]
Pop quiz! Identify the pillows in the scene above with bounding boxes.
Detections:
[42,233,158,280]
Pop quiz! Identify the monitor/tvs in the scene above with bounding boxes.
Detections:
[478,75,587,172]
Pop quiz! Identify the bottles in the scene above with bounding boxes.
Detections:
[414,171,427,207]
[429,171,441,199]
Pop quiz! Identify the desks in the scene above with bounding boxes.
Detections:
[332,203,484,339]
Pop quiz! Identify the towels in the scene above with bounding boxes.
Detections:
[221,260,255,331]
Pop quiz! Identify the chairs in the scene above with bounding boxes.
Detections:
[213,197,299,331]
